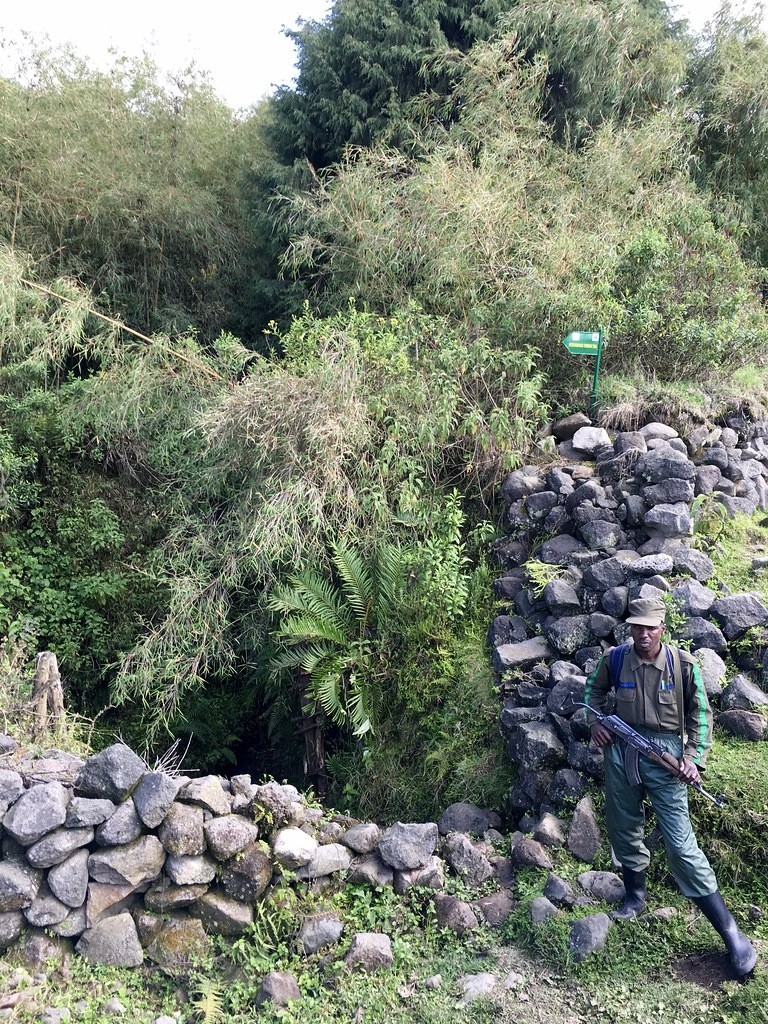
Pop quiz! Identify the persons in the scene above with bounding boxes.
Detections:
[584,598,757,975]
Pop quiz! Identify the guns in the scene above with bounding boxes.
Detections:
[557,689,728,812]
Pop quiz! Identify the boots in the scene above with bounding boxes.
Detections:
[691,887,756,976]
[608,864,647,923]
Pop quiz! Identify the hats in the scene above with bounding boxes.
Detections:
[626,597,667,626]
